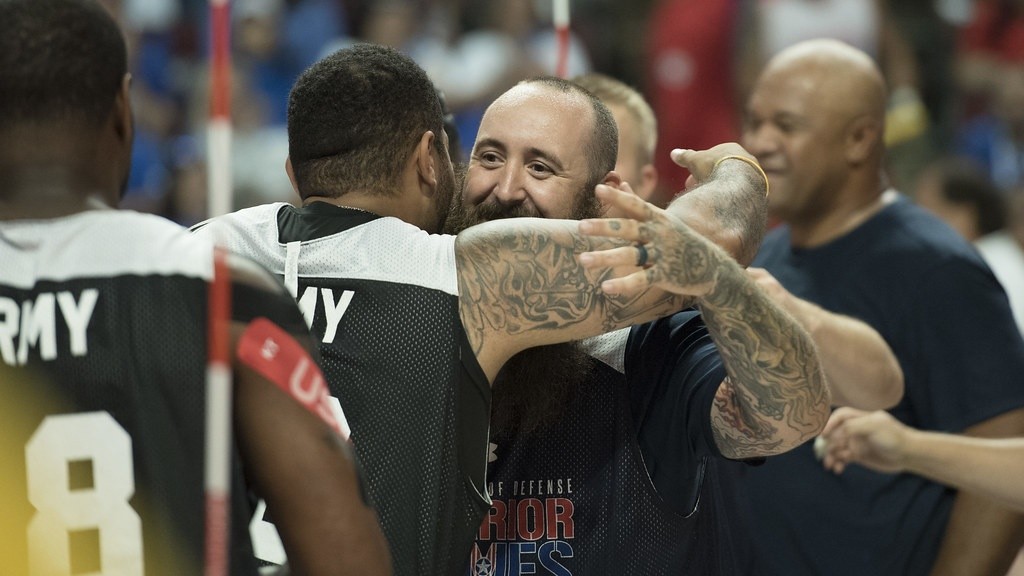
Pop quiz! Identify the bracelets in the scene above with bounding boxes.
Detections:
[714,155,769,198]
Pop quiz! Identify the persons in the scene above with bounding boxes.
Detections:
[99,0,1023,576]
[447,75,830,576]
[0,0,394,576]
[182,44,768,576]
